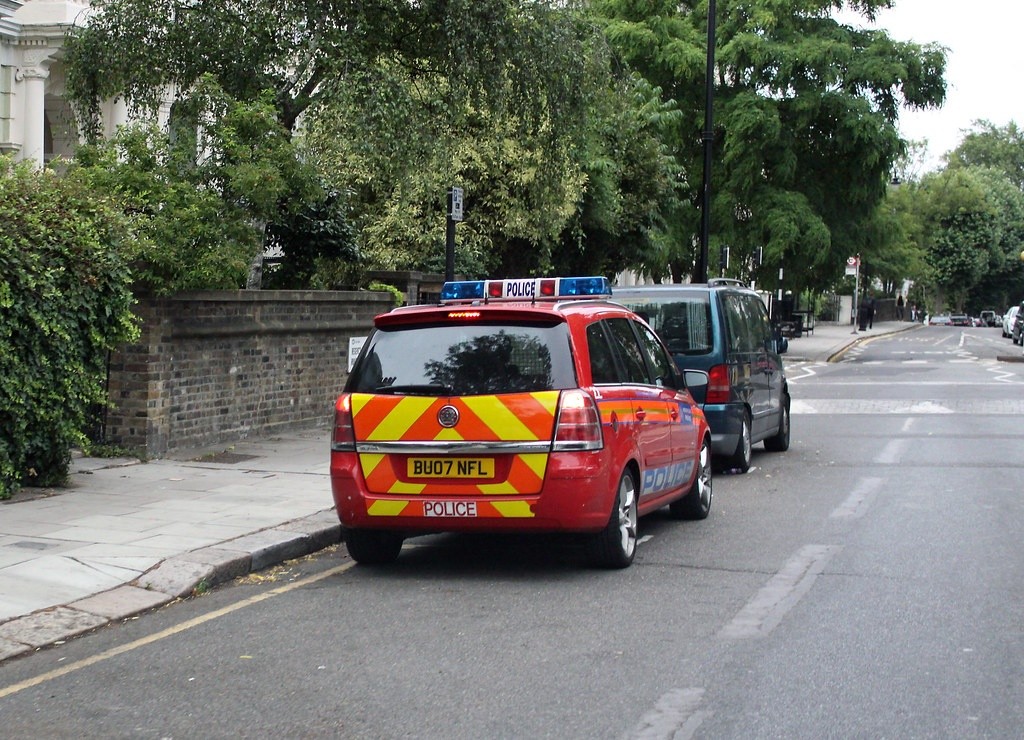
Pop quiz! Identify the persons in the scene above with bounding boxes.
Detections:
[897,296,904,322]
[866,291,875,329]
[911,305,918,322]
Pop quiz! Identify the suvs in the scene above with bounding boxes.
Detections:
[569,278,791,474]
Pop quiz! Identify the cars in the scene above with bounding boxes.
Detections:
[792,313,815,331]
[1002,300,1024,346]
[330,276,714,569]
[929,310,1003,328]
